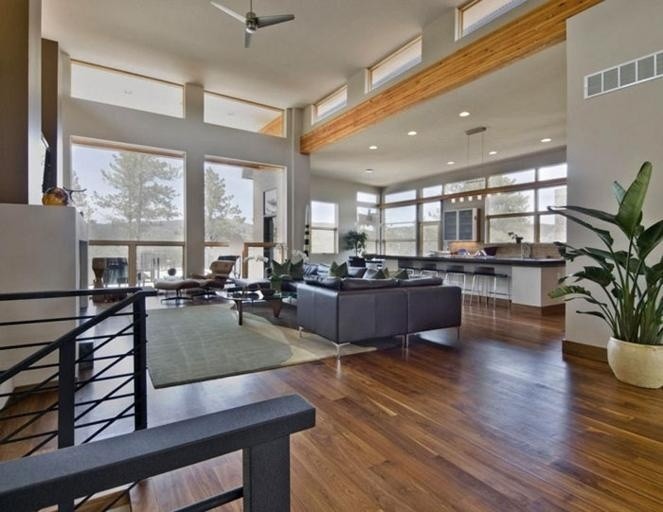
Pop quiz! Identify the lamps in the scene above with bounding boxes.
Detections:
[444,126,502,205]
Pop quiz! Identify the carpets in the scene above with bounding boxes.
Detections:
[145,299,376,391]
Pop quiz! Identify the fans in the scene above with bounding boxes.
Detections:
[206,0,295,49]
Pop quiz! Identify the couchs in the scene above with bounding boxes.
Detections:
[233,262,409,308]
[295,277,463,358]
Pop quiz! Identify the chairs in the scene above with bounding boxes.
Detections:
[154,256,238,300]
[397,258,418,277]
[418,263,446,278]
[470,266,509,315]
[443,264,472,304]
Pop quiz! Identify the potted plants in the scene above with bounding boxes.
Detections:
[544,161,662,389]
[343,229,367,267]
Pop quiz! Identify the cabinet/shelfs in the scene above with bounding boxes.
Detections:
[443,208,480,242]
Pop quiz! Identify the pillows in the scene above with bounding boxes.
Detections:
[271,257,409,280]
